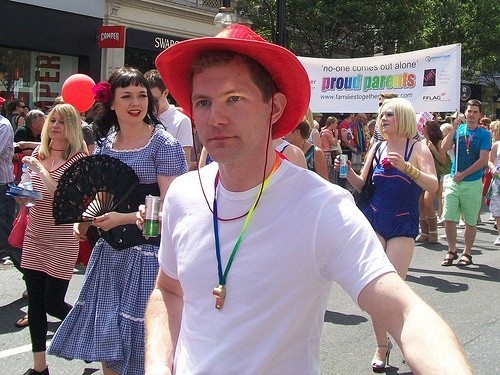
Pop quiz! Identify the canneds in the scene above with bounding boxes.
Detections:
[142,194,162,238]
[338,154,349,178]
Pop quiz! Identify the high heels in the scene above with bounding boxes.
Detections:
[370,338,392,369]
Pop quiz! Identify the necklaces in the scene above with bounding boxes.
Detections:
[52,159,55,166]
[51,148,64,151]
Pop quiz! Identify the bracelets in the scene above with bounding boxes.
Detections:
[401,162,420,181]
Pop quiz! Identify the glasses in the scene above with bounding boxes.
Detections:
[318,116,323,118]
[480,123,487,126]
[17,105,26,109]
[154,90,164,103]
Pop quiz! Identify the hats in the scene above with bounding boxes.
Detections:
[156,22,310,140]
[313,114,323,117]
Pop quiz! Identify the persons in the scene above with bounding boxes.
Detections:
[145,25,472,375]
[415,120,446,244]
[46,67,189,375]
[0,96,97,272]
[198,145,215,168]
[370,93,422,146]
[145,70,193,168]
[334,98,439,370]
[441,100,492,268]
[271,135,308,174]
[300,106,500,244]
[284,119,327,182]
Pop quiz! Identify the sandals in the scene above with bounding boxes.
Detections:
[457,253,473,265]
[441,251,458,266]
[15,315,29,327]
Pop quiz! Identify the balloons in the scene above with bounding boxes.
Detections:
[62,74,97,112]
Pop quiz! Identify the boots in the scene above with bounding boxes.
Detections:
[425,215,438,243]
[415,218,429,242]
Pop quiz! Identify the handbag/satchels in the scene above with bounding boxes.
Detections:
[330,142,342,154]
[482,171,493,196]
[434,154,452,176]
[8,203,29,248]
[485,186,494,206]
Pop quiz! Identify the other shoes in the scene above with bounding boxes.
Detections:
[489,212,496,220]
[23,366,49,375]
[437,220,444,226]
[494,222,498,229]
[494,237,500,245]
[22,291,28,298]
